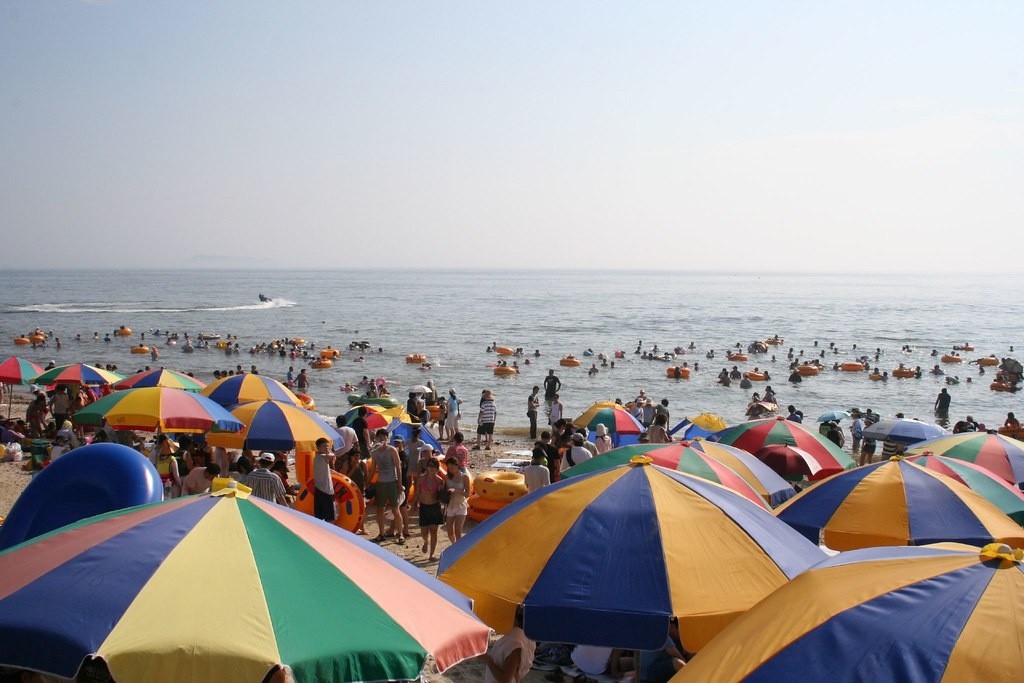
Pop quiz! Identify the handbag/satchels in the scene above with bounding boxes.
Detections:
[437,490,451,504]
[457,411,462,419]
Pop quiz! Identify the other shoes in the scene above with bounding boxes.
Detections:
[429,557,439,564]
[422,542,428,553]
[385,532,410,537]
[472,445,490,450]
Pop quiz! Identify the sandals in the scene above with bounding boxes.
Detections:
[397,535,405,544]
[572,674,597,683]
[371,535,385,542]
[542,669,564,682]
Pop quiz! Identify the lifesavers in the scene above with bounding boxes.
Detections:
[728,339,826,381]
[165,331,447,421]
[292,468,366,535]
[493,367,517,376]
[496,346,515,355]
[998,426,1024,441]
[559,358,581,367]
[12,323,132,345]
[365,453,529,523]
[666,366,691,379]
[841,362,864,372]
[868,373,883,381]
[893,367,917,378]
[130,346,150,355]
[941,341,1022,393]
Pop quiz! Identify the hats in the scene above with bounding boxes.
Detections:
[570,433,584,442]
[394,435,404,442]
[533,447,548,458]
[595,423,609,436]
[57,430,68,436]
[416,444,433,451]
[482,390,495,400]
[261,453,275,462]
[449,388,455,393]
[162,438,181,455]
[30,385,39,392]
[637,389,652,404]
[281,465,289,473]
[50,360,55,363]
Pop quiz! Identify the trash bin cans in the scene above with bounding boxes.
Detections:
[30,439,51,472]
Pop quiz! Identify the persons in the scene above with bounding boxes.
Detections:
[0,325,1022,680]
